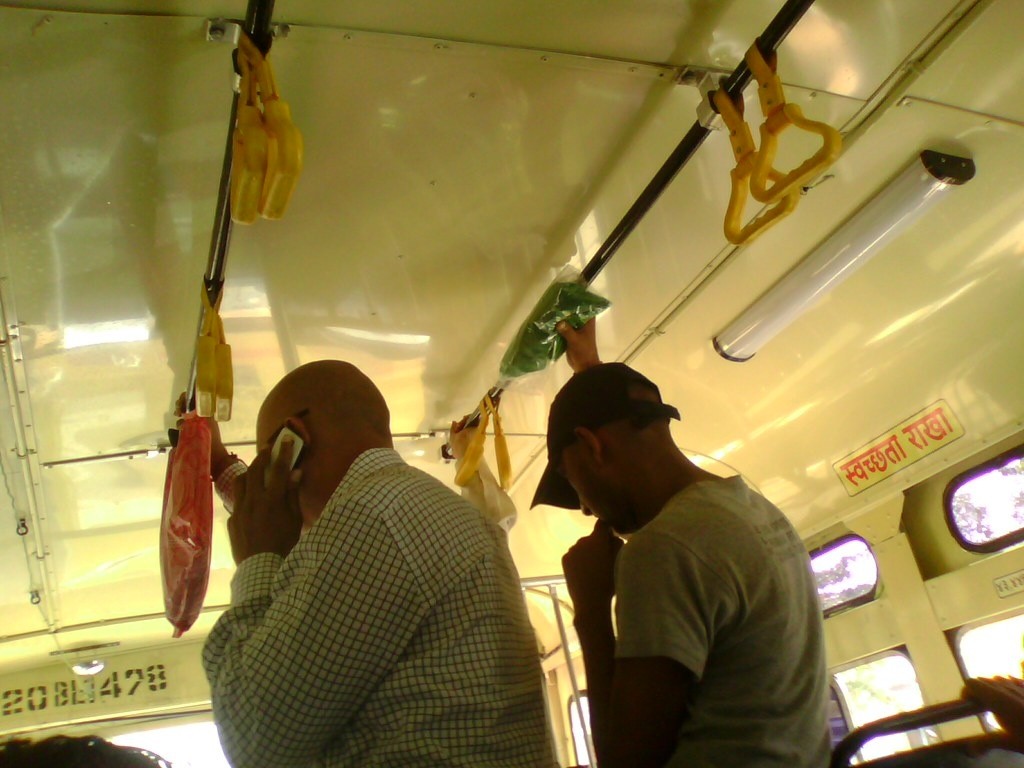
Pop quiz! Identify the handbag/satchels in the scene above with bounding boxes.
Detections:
[159,409,211,638]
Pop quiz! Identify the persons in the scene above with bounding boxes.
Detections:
[960,675,1024,756]
[0,734,162,768]
[532,316,837,768]
[173,359,560,768]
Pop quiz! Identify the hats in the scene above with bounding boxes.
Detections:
[528,362,680,511]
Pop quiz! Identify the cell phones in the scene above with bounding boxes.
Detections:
[262,427,304,487]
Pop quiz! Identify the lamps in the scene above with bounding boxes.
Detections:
[713,152,974,361]
